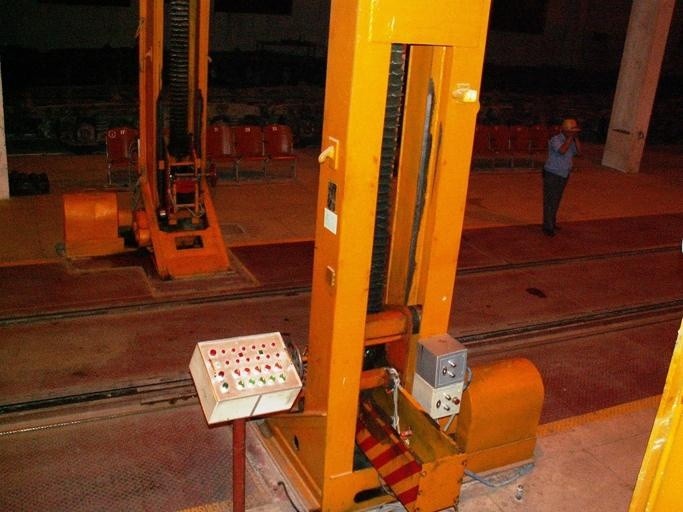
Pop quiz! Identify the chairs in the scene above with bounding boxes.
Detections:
[473,126,563,165]
[103,122,297,186]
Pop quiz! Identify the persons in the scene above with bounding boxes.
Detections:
[541,117,583,237]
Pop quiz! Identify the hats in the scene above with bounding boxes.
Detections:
[560,118,582,132]
